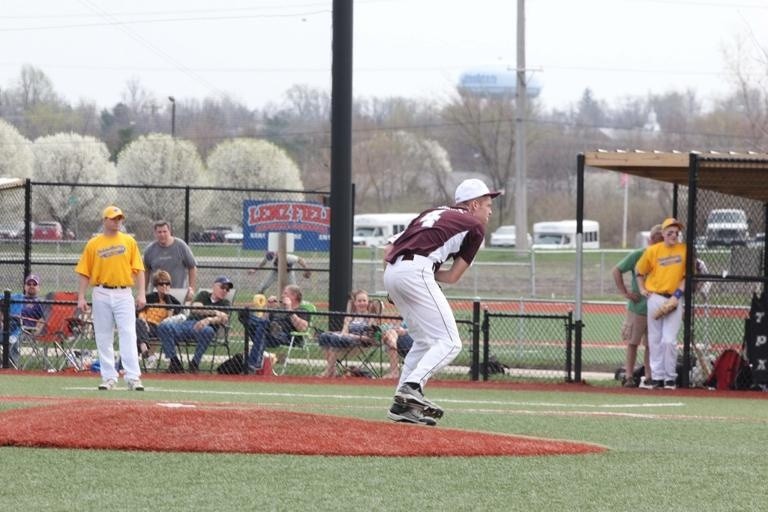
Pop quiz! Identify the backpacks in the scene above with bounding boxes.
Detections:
[715,349,744,389]
[217,351,247,376]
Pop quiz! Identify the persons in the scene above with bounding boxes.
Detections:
[382,179,502,427]
[131,270,414,379]
[635,218,687,392]
[142,221,199,304]
[0,275,47,362]
[74,207,146,391]
[248,252,311,294]
[694,256,712,308]
[614,224,663,387]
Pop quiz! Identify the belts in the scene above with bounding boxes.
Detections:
[659,293,673,298]
[96,282,129,290]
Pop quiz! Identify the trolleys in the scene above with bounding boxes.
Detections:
[612,291,715,386]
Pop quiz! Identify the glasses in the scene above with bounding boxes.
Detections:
[220,285,230,291]
[157,282,171,286]
[27,283,37,286]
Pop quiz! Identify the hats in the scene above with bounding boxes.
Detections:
[215,276,233,288]
[103,205,126,222]
[25,274,40,285]
[455,177,502,206]
[660,219,684,233]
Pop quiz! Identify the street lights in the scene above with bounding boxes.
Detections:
[165,94,180,139]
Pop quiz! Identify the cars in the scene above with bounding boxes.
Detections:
[701,205,755,250]
[0,219,77,243]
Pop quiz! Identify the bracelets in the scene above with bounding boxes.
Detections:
[672,288,683,299]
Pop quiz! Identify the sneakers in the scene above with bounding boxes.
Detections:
[98,378,117,391]
[128,379,145,391]
[387,401,438,427]
[171,359,185,373]
[187,359,200,372]
[623,374,678,390]
[393,384,445,420]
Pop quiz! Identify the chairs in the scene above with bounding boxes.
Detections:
[141,285,193,375]
[262,298,316,376]
[18,288,84,375]
[334,298,386,379]
[175,286,236,374]
[0,292,29,373]
